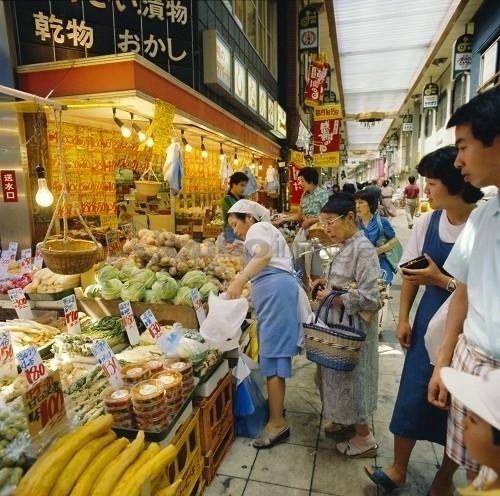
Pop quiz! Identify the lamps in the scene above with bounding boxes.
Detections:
[31,101,56,210]
[107,106,270,171]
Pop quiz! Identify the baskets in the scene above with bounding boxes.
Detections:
[134,181,163,196]
[37,239,102,275]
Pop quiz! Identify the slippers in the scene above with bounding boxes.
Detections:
[253,407,400,496]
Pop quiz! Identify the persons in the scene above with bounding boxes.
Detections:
[425,81,500,495]
[438,366,500,496]
[313,193,382,459]
[225,198,313,450]
[362,144,483,495]
[215,168,420,339]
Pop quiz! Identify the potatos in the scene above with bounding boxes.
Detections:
[121,228,242,280]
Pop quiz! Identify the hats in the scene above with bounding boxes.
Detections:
[440,366,499,431]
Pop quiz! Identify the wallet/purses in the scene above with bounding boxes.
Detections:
[400,256,428,275]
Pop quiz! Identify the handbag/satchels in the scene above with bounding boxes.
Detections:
[303,291,366,372]
[376,235,403,273]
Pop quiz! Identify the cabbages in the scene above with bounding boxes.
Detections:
[82,258,219,308]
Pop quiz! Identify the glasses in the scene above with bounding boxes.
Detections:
[317,211,348,230]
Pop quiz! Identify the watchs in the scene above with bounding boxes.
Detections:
[447,277,457,293]
